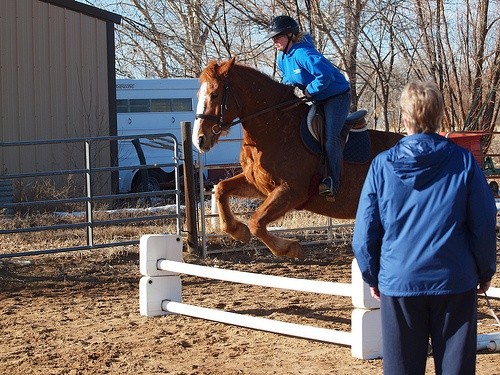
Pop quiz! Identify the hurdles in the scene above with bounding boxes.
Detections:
[140,233,384,358]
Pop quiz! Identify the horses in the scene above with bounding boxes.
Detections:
[191,56,409,261]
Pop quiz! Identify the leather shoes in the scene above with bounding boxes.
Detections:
[319,176,337,201]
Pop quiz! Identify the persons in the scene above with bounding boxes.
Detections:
[263,15,351,202]
[351,79,497,375]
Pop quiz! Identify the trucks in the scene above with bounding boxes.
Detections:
[116,78,243,200]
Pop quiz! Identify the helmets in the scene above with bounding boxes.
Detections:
[265,16,299,38]
